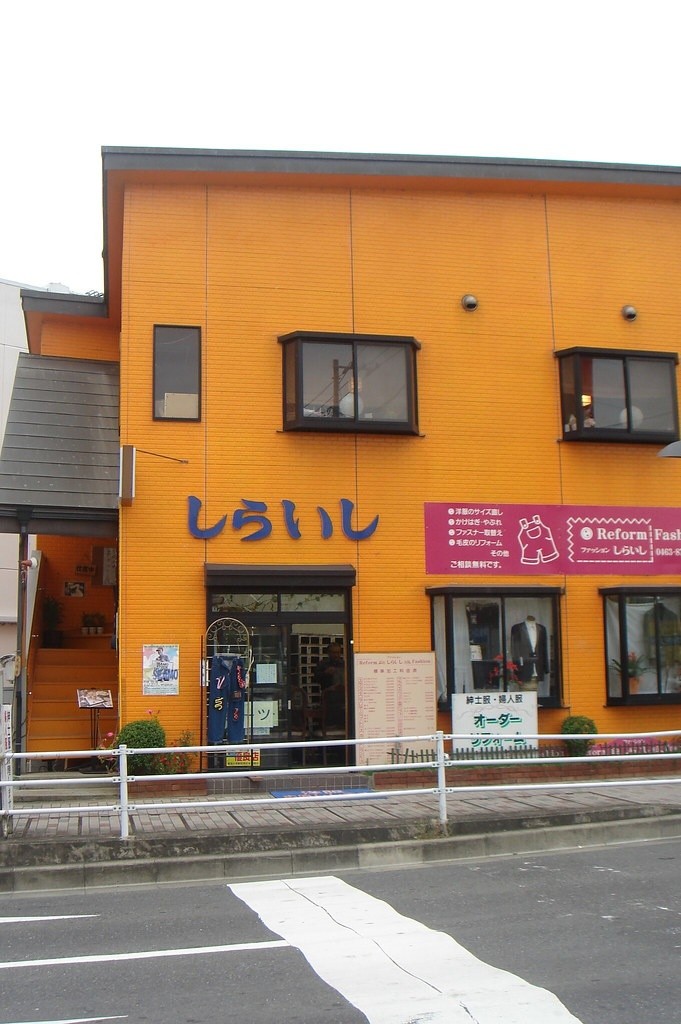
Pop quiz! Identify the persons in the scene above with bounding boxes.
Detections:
[156,648,168,661]
[313,643,345,728]
[510,617,549,691]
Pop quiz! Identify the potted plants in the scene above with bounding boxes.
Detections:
[40,596,105,647]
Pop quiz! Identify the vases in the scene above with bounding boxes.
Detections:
[618,675,639,695]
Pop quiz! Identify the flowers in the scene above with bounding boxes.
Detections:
[610,652,656,677]
